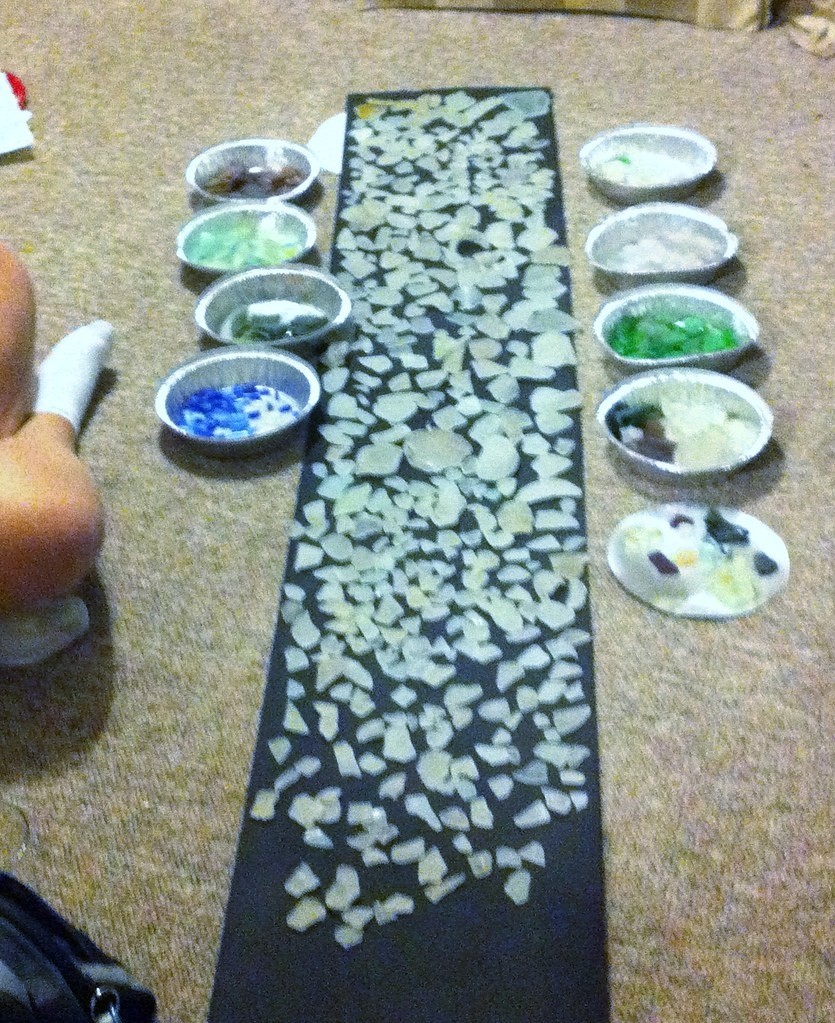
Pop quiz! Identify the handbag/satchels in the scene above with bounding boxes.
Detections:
[0,872,158,1023]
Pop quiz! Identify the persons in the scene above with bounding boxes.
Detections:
[0,243,110,610]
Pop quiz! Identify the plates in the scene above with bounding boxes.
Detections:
[607,501,790,616]
